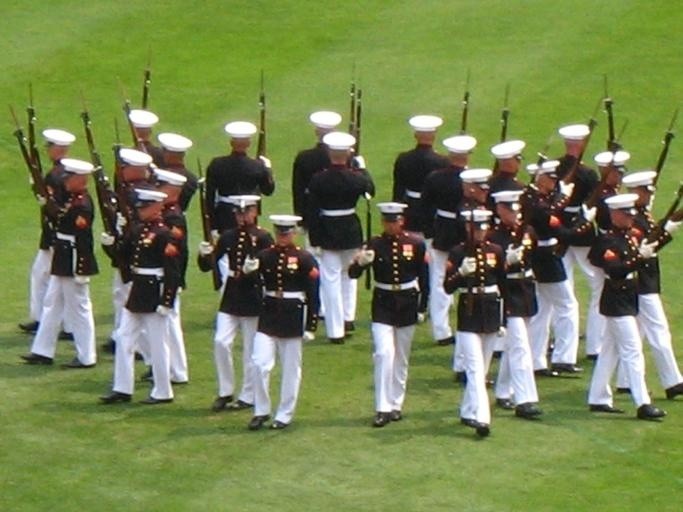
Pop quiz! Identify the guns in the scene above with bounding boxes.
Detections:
[526,144,549,187]
[649,107,678,188]
[604,74,616,153]
[667,207,683,222]
[117,75,149,158]
[350,80,355,136]
[8,102,56,225]
[466,201,475,315]
[242,204,263,312]
[26,83,43,189]
[78,91,116,241]
[514,174,538,247]
[354,87,361,154]
[366,193,371,289]
[552,158,616,257]
[141,46,152,111]
[461,64,470,135]
[499,84,510,143]
[611,120,628,154]
[644,185,683,244]
[562,99,603,184]
[258,68,267,157]
[197,158,223,291]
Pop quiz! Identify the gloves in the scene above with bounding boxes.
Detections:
[560,180,575,198]
[241,253,260,273]
[355,249,375,267]
[302,330,315,343]
[638,238,659,259]
[504,242,526,267]
[582,203,598,222]
[498,326,507,336]
[101,234,115,247]
[417,313,425,323]
[457,255,477,275]
[354,154,366,170]
[115,212,126,233]
[199,241,213,259]
[259,155,271,168]
[664,219,681,233]
[156,305,170,317]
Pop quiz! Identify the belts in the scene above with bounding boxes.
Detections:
[406,189,421,198]
[217,194,228,204]
[436,208,456,219]
[322,208,356,217]
[564,206,580,213]
[537,236,558,247]
[131,238,558,299]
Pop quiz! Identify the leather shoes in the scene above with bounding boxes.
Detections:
[214,396,253,410]
[331,337,343,344]
[346,321,354,331]
[19,322,187,404]
[439,336,683,436]
[270,421,287,428]
[249,415,268,430]
[372,411,400,426]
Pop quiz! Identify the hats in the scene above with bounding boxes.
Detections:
[490,190,526,210]
[149,167,188,188]
[270,214,303,231]
[128,110,159,129]
[230,194,261,208]
[322,131,357,151]
[157,132,193,153]
[408,115,443,133]
[605,193,640,216]
[461,209,494,230]
[460,168,494,191]
[621,170,656,194]
[134,189,167,209]
[118,147,154,170]
[44,128,75,147]
[225,121,258,140]
[442,135,477,155]
[594,150,631,168]
[310,111,342,132]
[491,139,526,161]
[526,160,560,178]
[558,124,592,143]
[60,157,96,179]
[377,202,409,222]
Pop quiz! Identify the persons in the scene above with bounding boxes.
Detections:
[292,110,342,321]
[445,125,630,394]
[15,128,76,332]
[99,110,198,384]
[303,131,375,345]
[617,171,683,397]
[588,193,667,418]
[419,135,476,346]
[392,115,444,315]
[205,121,274,299]
[348,202,430,427]
[100,188,187,404]
[443,210,509,437]
[241,215,321,430]
[19,157,97,369]
[198,195,275,410]
[487,189,544,418]
